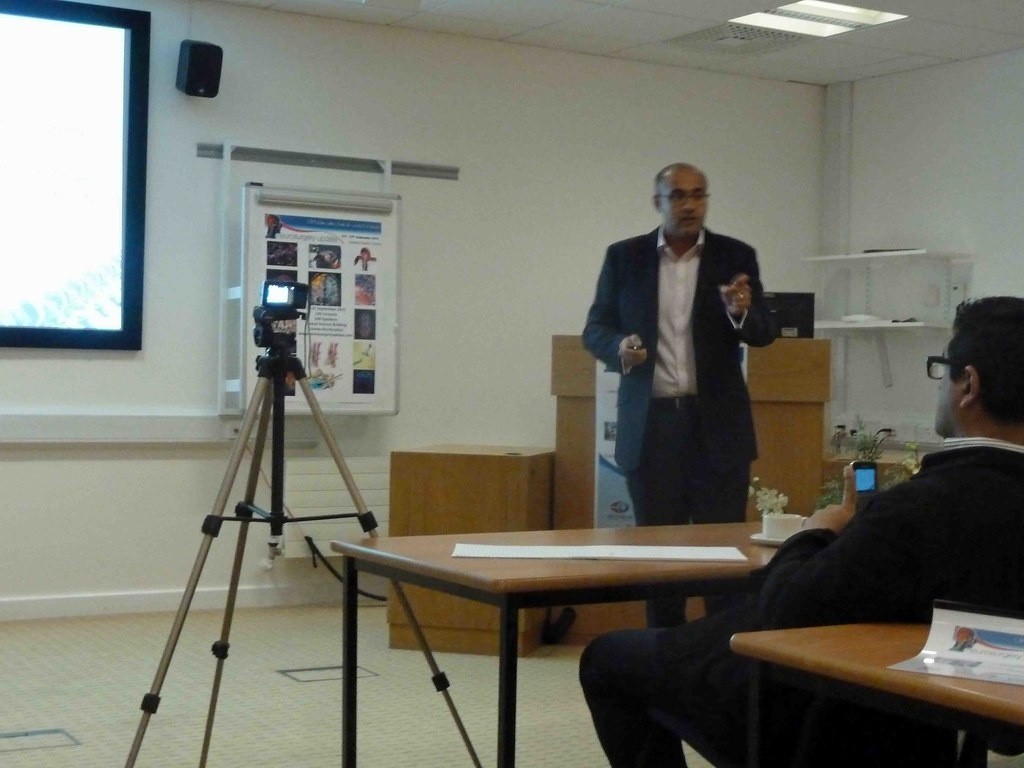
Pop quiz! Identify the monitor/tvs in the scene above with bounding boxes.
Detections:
[762,290,815,339]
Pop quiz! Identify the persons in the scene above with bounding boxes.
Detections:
[581,162,783,628]
[578,297,1024,768]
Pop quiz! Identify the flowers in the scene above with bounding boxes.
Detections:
[748,477,788,516]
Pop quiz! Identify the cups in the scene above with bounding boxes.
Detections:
[763,513,809,538]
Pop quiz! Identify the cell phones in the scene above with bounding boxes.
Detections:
[848,457,879,516]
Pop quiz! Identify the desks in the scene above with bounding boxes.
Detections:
[730,623,1024,768]
[330,522,783,768]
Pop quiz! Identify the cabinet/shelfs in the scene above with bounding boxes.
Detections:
[802,248,971,388]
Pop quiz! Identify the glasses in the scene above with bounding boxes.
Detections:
[926,355,967,380]
[653,190,709,203]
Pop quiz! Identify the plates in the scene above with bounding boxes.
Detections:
[751,532,786,547]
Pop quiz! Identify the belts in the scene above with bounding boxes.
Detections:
[651,396,698,411]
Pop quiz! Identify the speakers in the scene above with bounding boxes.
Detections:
[173,38,224,99]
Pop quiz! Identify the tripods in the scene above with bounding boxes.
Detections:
[125,312,482,767]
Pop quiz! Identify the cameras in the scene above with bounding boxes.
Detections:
[258,278,309,309]
